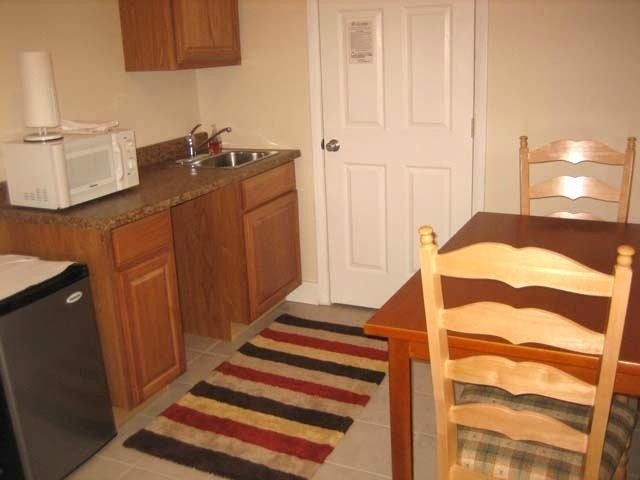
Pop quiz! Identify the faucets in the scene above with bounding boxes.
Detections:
[186,123,232,153]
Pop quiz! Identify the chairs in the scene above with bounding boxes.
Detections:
[519,134,638,224]
[418,224,638,480]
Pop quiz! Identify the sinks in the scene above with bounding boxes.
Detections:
[199,150,271,169]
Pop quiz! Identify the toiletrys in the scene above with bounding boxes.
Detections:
[211,122,223,153]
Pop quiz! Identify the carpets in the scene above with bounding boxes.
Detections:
[122,314,388,480]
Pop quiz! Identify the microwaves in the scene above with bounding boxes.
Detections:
[3,130,141,211]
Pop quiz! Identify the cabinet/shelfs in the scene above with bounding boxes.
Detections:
[170,160,304,343]
[118,1,242,73]
[0,207,189,413]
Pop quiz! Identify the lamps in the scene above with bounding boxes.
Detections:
[19,51,64,142]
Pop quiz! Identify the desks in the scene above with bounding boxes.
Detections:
[362,209,637,480]
